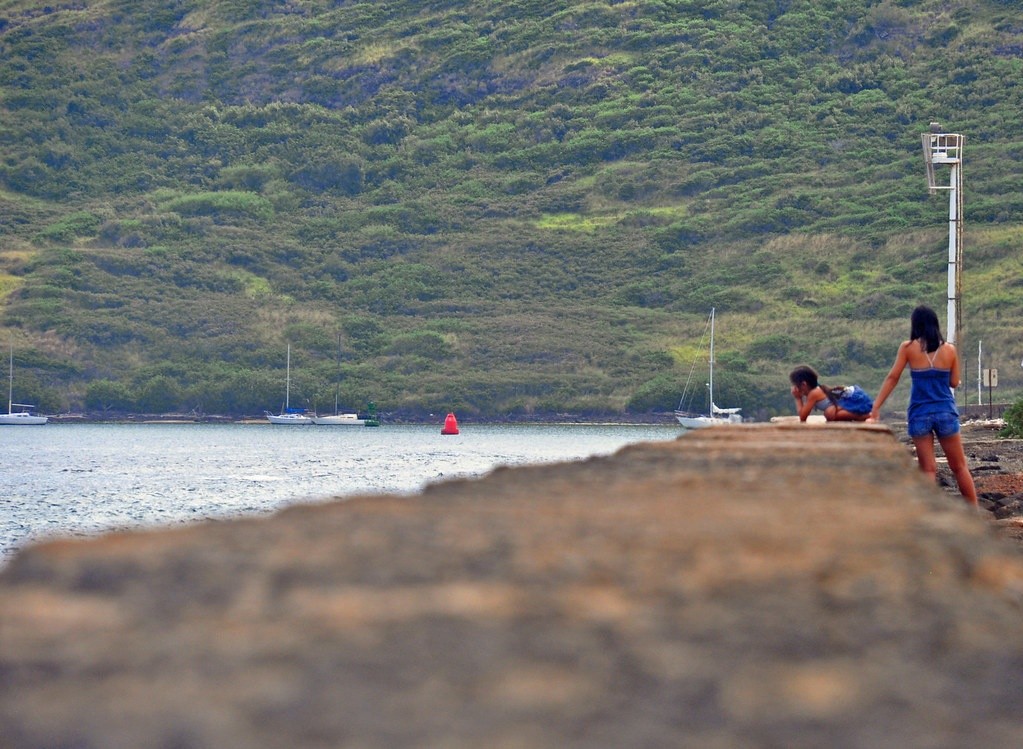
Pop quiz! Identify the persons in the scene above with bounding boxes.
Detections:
[873,305,978,509]
[790,365,873,422]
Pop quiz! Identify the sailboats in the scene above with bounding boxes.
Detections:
[674,306,745,429]
[313,334,374,425]
[264,343,314,425]
[0,345,50,426]
